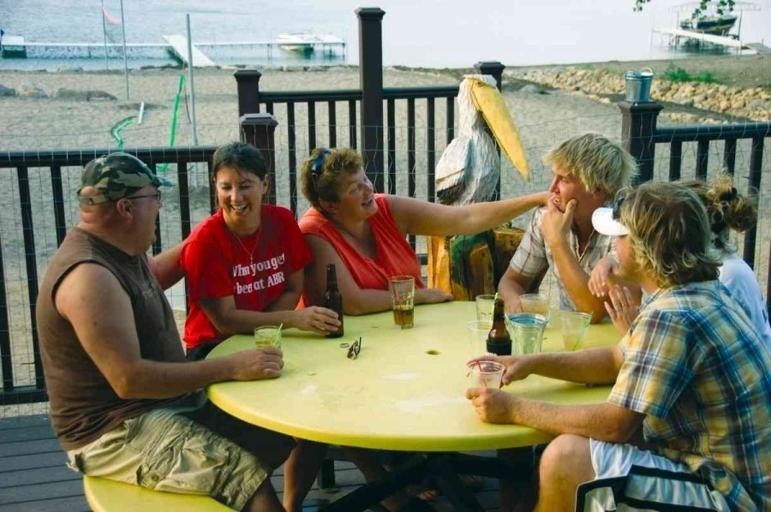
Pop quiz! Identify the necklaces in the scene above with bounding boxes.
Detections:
[237,232,259,267]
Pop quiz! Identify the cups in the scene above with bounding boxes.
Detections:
[467,292,593,356]
[467,360,505,389]
[389,275,415,329]
[255,325,280,351]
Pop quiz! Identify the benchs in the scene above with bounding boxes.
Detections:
[83,473,239,512]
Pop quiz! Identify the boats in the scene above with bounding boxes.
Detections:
[681,0,737,34]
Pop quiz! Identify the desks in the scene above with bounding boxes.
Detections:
[203,300,627,451]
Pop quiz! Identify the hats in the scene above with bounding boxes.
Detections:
[591,206,634,237]
[75,151,175,206]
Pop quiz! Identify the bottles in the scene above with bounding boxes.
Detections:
[323,263,345,339]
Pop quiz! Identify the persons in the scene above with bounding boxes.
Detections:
[464,181,771,512]
[586,173,770,354]
[296,146,554,503]
[34,152,292,512]
[179,142,342,512]
[497,131,641,325]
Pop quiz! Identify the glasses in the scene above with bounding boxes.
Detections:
[347,336,363,361]
[612,197,629,226]
[311,147,334,198]
[125,190,163,202]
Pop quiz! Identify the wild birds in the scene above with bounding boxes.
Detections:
[435,74,532,288]
